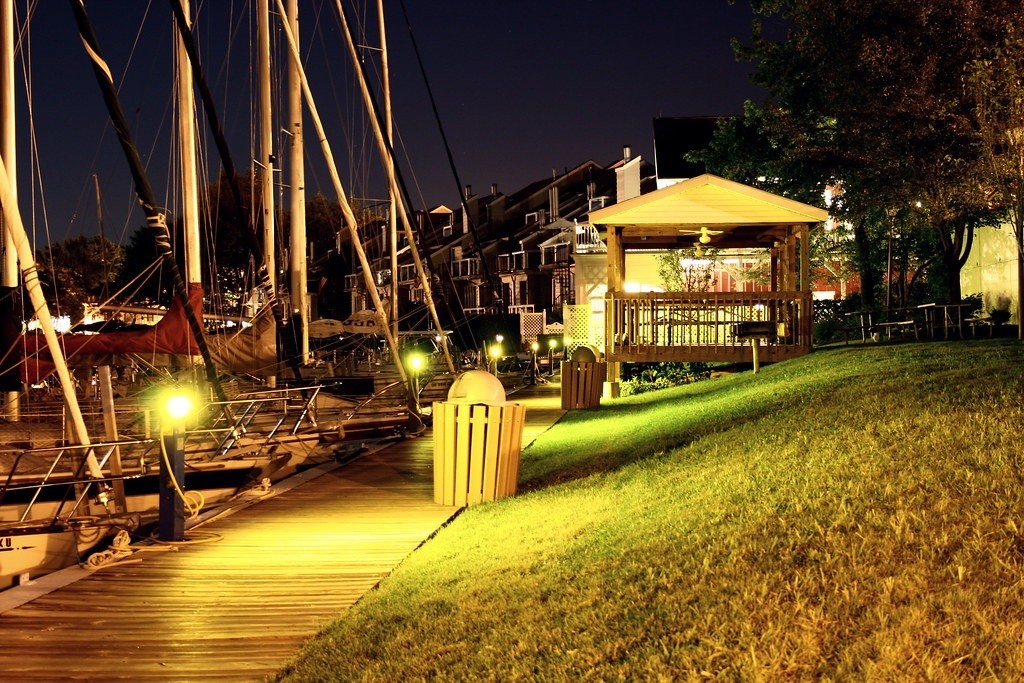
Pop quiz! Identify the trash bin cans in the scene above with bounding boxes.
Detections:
[431,369,527,510]
[556,345,608,411]
[585,343,606,362]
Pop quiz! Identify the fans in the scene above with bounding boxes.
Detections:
[678,226,732,243]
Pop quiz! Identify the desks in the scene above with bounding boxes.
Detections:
[844,309,914,344]
[917,303,970,340]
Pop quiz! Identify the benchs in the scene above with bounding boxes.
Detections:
[842,318,1003,345]
